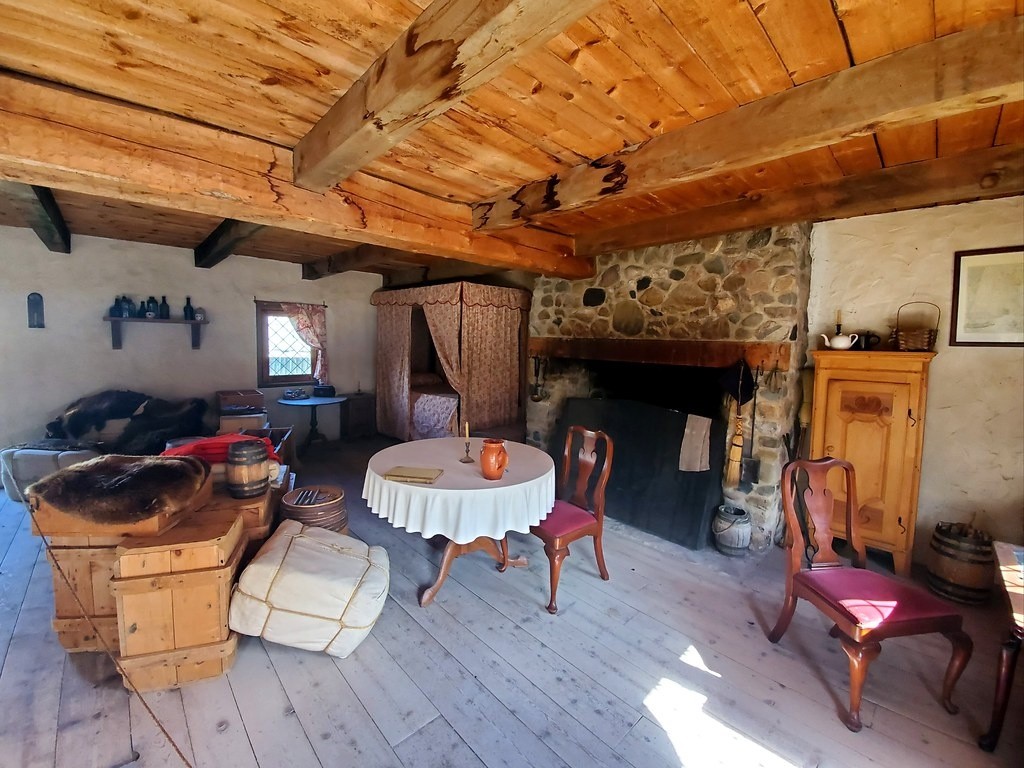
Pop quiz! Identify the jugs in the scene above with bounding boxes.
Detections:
[855,331,881,350]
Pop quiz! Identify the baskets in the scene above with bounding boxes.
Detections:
[892,301,942,353]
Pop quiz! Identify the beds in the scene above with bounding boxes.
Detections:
[370,281,531,442]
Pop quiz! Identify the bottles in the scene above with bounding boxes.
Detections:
[147,297,158,318]
[159,296,170,319]
[183,297,194,321]
[480,438,507,480]
[137,302,147,318]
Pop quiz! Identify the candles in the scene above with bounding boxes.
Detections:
[465,421,469,442]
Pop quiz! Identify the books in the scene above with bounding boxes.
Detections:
[384,466,444,484]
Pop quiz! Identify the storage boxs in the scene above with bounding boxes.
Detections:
[240,427,296,469]
[217,390,263,414]
[28,470,214,537]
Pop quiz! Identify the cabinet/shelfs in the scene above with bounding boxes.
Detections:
[805,351,939,578]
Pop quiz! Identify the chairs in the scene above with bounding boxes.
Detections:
[768,457,974,734]
[495,425,614,613]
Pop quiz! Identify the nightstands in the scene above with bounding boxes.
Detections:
[340,392,377,442]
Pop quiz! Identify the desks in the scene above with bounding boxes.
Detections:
[362,437,555,607]
[978,541,1024,752]
[277,396,347,448]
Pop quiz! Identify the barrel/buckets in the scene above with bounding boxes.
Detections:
[923,521,997,606]
[280,484,347,542]
[710,503,751,556]
[227,439,270,498]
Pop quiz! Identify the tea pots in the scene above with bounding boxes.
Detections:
[820,333,859,350]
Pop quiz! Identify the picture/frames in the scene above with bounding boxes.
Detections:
[949,245,1024,347]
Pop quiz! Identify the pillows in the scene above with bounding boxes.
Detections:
[411,373,442,386]
